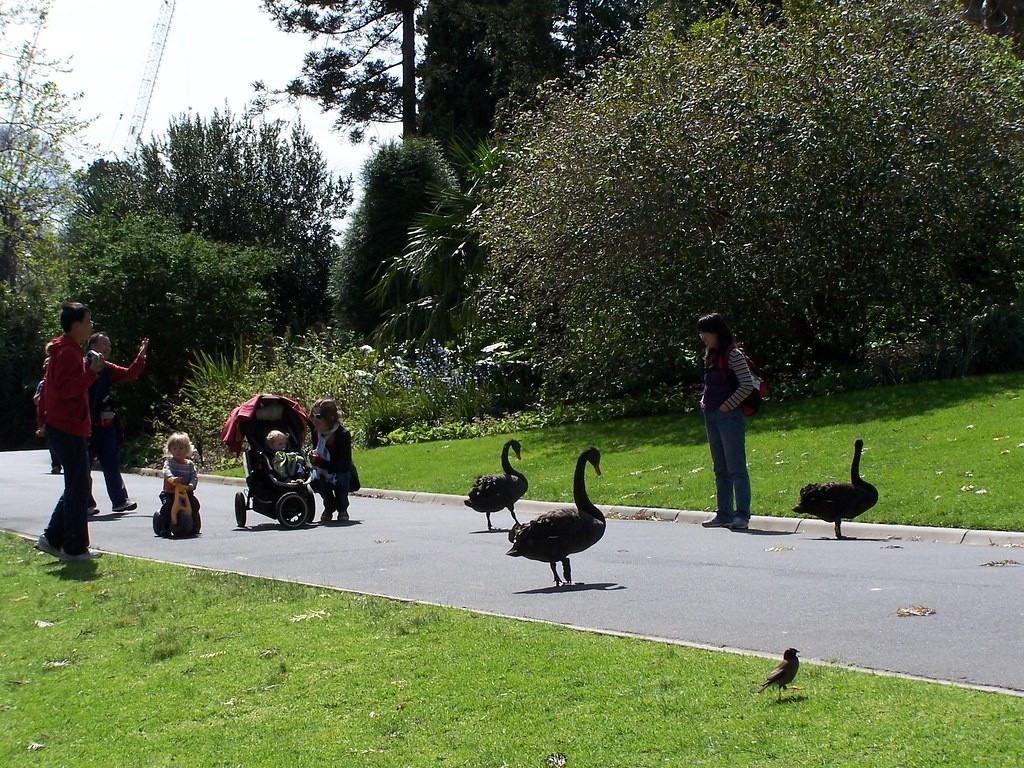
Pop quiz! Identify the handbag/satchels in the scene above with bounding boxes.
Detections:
[717,343,765,417]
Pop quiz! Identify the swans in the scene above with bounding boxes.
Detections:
[463,438,529,532]
[505,446,606,586]
[791,439,879,540]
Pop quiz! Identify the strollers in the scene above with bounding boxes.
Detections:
[220,394,317,530]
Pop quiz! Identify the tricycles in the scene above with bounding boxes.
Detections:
[153,482,201,540]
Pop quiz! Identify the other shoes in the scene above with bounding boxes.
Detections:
[338,510,349,522]
[320,507,336,521]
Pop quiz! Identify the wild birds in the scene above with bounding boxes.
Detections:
[753,648,801,700]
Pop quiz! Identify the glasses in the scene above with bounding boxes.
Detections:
[310,411,323,421]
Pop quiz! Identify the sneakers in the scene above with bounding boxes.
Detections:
[88,506,100,514]
[33,532,62,557]
[58,549,103,561]
[701,518,732,527]
[724,519,749,529]
[112,498,138,511]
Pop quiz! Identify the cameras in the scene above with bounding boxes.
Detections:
[86,350,101,362]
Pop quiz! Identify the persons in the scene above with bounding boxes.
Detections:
[33,332,149,515]
[158,431,202,537]
[696,312,754,529]
[32,301,106,561]
[309,398,352,523]
[262,430,306,484]
